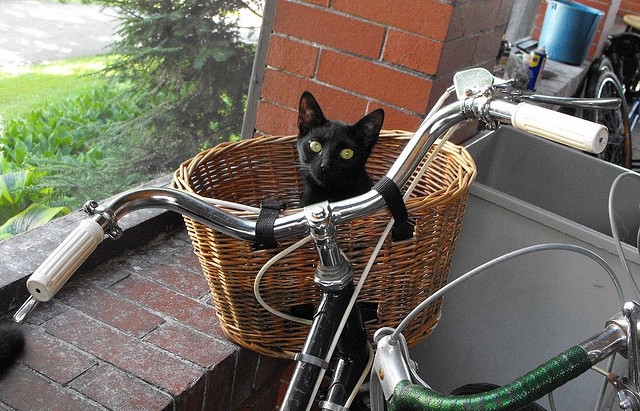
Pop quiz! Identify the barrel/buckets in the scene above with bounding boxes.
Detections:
[537,0,605,65]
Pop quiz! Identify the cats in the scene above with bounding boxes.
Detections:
[289,89,385,206]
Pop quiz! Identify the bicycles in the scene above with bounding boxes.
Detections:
[361,166,640,411]
[9,68,640,411]
[582,67,640,172]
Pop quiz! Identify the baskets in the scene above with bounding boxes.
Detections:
[170,127,478,362]
